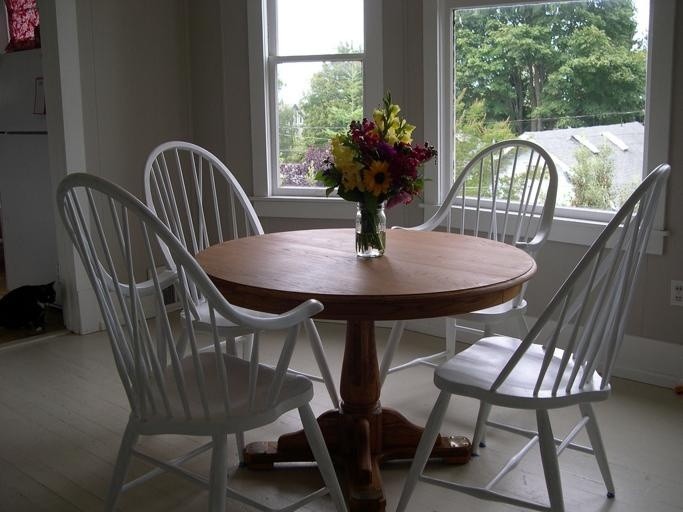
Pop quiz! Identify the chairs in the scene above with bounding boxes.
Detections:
[379,137,559,448]
[55,171,348,512]
[144,140,341,409]
[396,163,671,510]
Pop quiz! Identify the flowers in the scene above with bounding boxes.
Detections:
[315,92,439,257]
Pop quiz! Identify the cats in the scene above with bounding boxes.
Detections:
[0,281,57,333]
